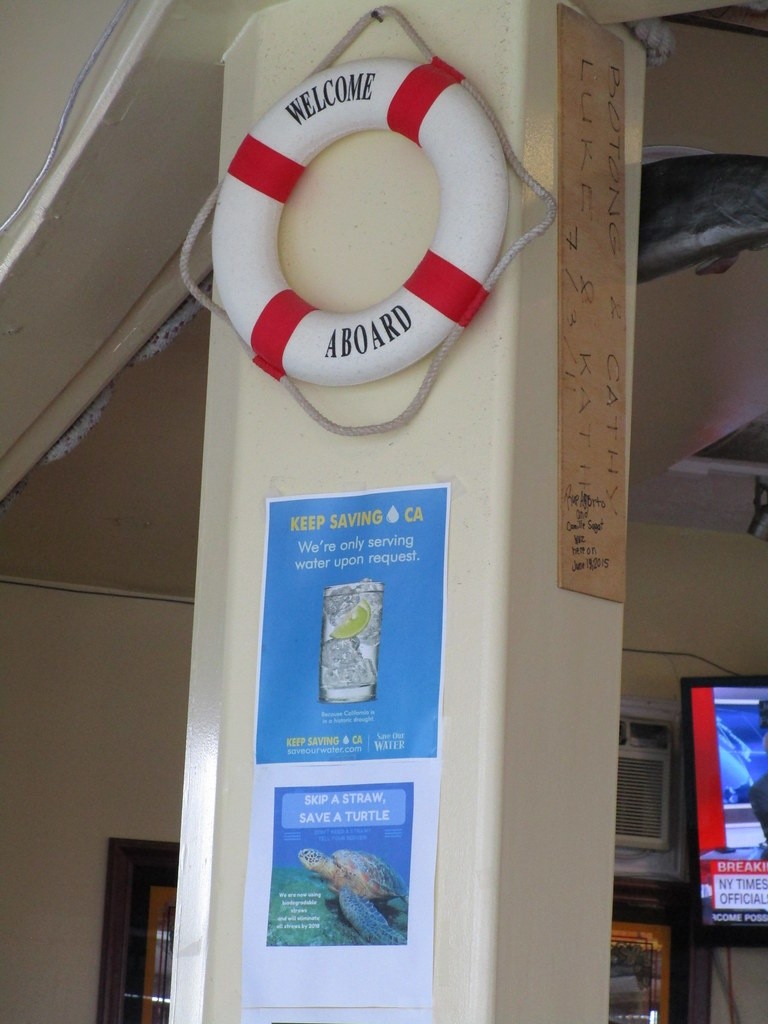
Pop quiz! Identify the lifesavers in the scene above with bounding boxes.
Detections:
[213,55,508,384]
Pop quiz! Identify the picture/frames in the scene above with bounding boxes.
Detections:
[96,837,713,1024]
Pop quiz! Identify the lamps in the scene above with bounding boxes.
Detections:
[747,474,768,543]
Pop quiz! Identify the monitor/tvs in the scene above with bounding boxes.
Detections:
[680,674,768,948]
